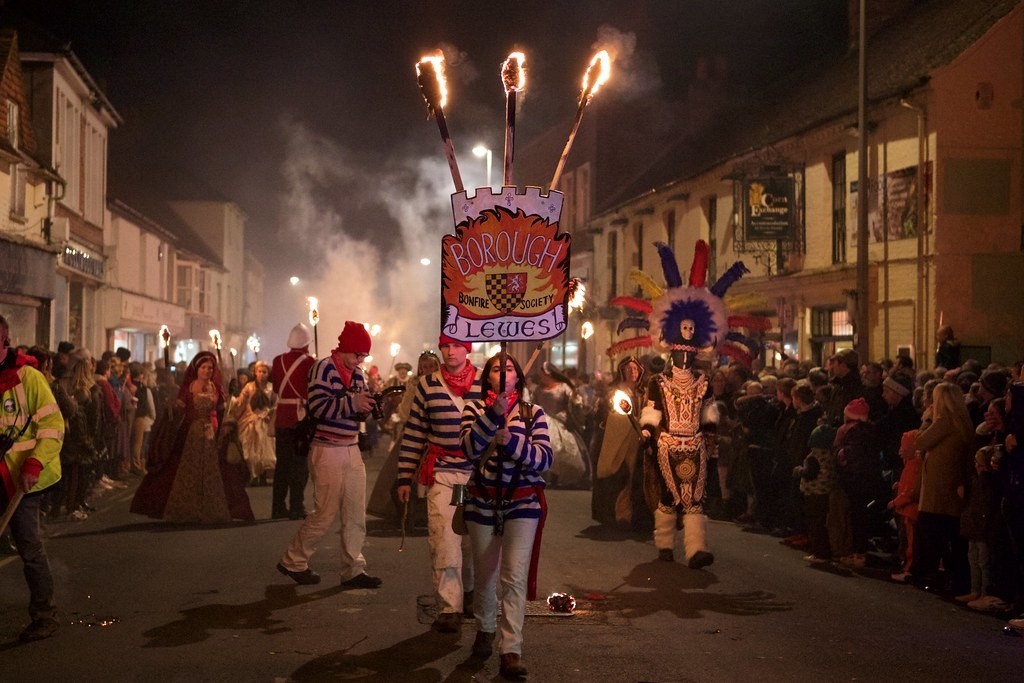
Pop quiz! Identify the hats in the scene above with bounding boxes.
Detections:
[843,398,870,422]
[339,321,371,355]
[57,341,75,354]
[809,425,837,448]
[395,362,413,372]
[438,332,472,355]
[883,372,912,396]
[116,347,130,362]
[980,369,1008,397]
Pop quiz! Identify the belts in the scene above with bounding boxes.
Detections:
[277,398,308,405]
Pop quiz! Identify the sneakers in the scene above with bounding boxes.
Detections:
[460,588,477,622]
[472,630,496,659]
[433,612,464,634]
[499,652,529,675]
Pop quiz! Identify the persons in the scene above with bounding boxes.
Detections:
[389,332,482,633]
[456,352,555,675]
[130,351,256,525]
[277,321,382,589]
[638,349,720,567]
[0,315,65,640]
[15,322,1024,629]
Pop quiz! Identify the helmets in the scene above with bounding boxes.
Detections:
[287,322,309,349]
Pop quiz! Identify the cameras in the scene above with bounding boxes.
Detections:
[368,395,384,419]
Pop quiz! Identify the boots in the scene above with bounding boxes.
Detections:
[271,480,290,519]
[289,480,308,520]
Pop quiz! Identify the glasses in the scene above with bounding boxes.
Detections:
[353,351,369,359]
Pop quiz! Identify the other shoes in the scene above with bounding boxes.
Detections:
[706,500,1024,632]
[689,552,714,569]
[276,564,320,582]
[342,572,383,588]
[658,547,673,562]
[18,619,54,641]
